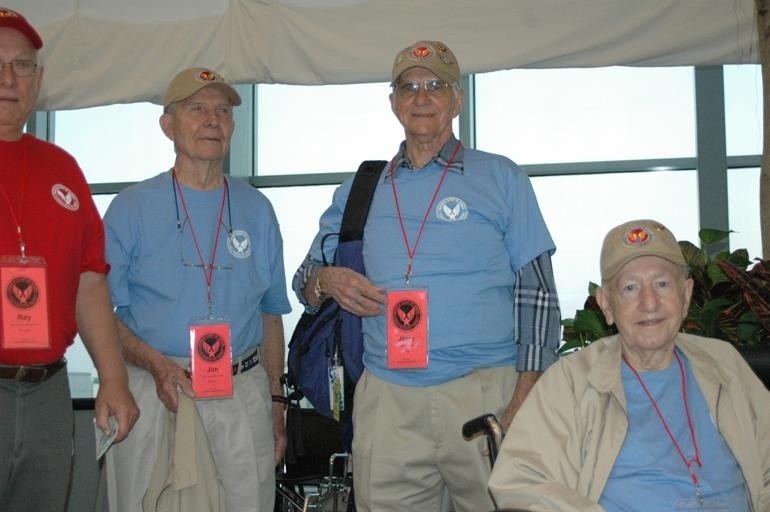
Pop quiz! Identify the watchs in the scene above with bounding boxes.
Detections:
[312,271,327,300]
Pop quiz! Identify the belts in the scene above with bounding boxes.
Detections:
[181,347,260,379]
[1,358,67,383]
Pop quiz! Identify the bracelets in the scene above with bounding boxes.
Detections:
[271,392,290,404]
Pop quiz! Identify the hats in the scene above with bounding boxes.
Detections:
[163,67,242,109]
[388,40,461,89]
[599,218,687,281]
[0,7,43,50]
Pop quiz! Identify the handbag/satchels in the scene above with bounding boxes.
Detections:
[286,237,367,421]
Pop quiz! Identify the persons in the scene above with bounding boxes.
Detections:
[292,40,561,512]
[104,67,293,512]
[0,4,141,512]
[487,220,770,512]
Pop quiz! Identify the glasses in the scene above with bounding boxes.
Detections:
[391,79,455,95]
[1,58,40,77]
